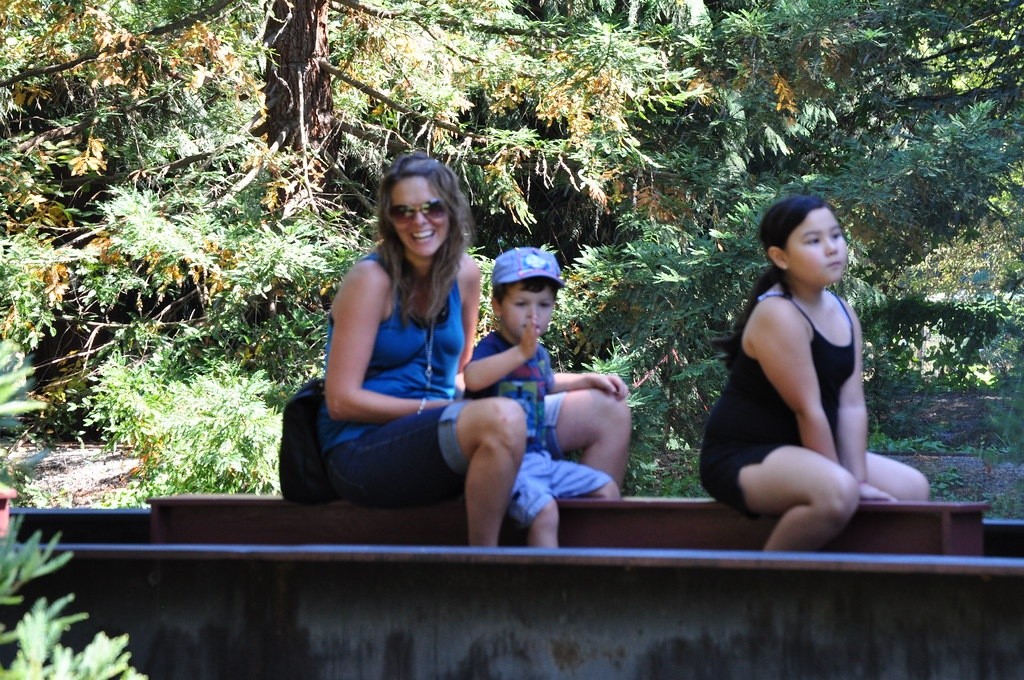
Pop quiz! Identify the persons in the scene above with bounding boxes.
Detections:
[700,196,930,553]
[323,154,632,549]
[463,246,628,548]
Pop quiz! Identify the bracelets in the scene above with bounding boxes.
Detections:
[417,398,427,414]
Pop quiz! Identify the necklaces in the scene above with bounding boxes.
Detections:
[424,317,436,387]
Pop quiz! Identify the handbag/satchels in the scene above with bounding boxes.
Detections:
[279,377,332,506]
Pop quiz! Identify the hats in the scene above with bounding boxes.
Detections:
[492,246,565,287]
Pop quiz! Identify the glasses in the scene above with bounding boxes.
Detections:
[386,198,446,223]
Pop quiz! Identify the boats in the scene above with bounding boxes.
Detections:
[0,495,1024,680]
